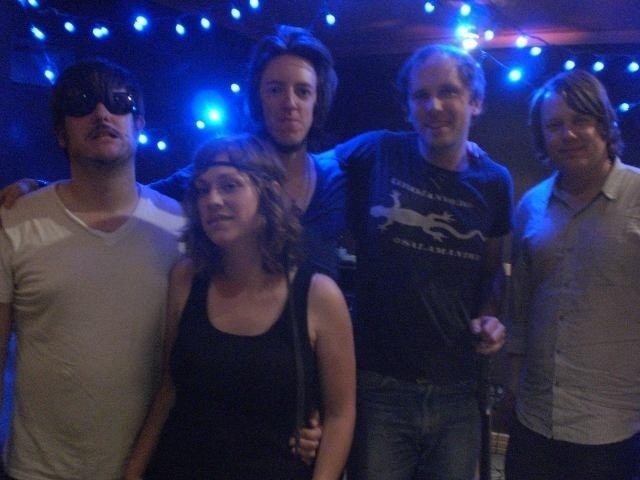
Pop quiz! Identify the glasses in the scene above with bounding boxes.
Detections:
[64,90,137,118]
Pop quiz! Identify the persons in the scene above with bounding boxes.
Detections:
[317,42,514,479]
[0,55,191,479]
[503,68,640,479]
[1,24,349,271]
[122,132,358,479]
